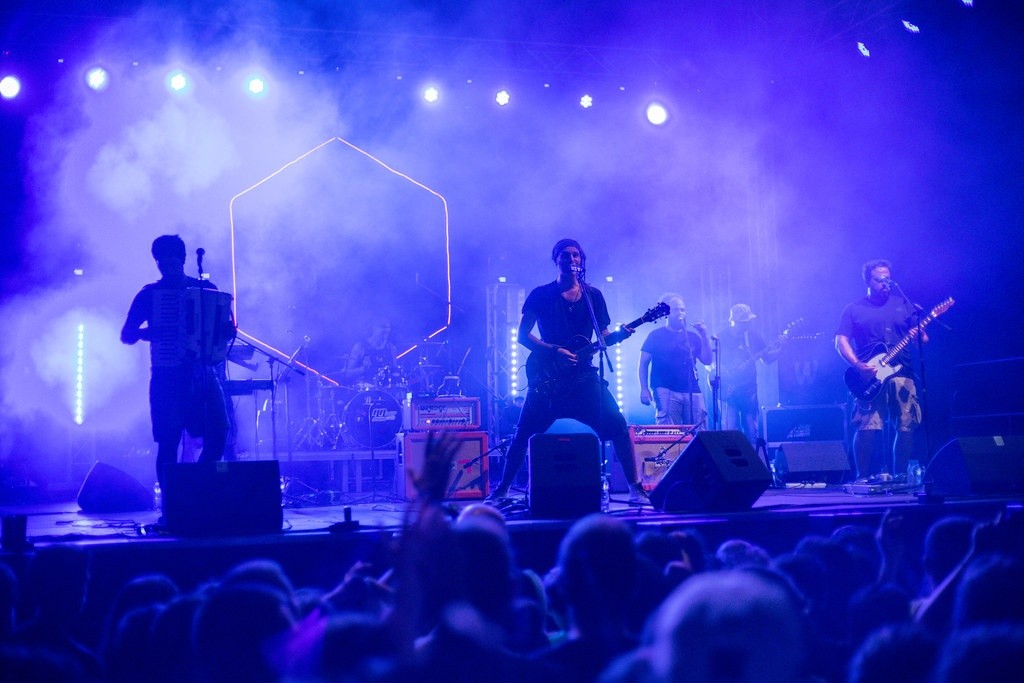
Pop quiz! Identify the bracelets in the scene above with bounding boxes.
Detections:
[549,344,561,357]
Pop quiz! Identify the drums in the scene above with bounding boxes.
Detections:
[341,388,403,448]
[317,385,349,421]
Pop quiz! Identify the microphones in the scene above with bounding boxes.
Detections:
[711,336,719,341]
[644,457,660,462]
[195,248,205,265]
[569,264,586,272]
[888,280,898,285]
[463,462,473,468]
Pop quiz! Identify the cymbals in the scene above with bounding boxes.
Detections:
[421,364,440,367]
[402,342,446,345]
[303,371,340,380]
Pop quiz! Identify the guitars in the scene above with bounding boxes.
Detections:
[844,297,955,404]
[706,317,806,402]
[524,301,671,397]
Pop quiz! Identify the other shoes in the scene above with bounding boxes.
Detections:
[627,482,651,504]
[482,480,511,506]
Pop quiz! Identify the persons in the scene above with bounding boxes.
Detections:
[120,234,238,524]
[704,304,789,461]
[0,428,1024,683]
[638,292,714,433]
[834,260,930,481]
[485,238,653,506]
[343,315,401,387]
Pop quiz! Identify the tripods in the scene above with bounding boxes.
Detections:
[282,342,358,507]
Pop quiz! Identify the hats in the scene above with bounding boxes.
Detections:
[728,303,757,322]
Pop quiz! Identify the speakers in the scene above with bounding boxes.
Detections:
[649,430,773,514]
[168,460,284,537]
[628,423,696,492]
[923,436,1024,500]
[528,433,601,521]
[395,430,490,501]
[774,442,851,489]
[77,461,156,516]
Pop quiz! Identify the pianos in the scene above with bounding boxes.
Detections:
[223,344,277,461]
